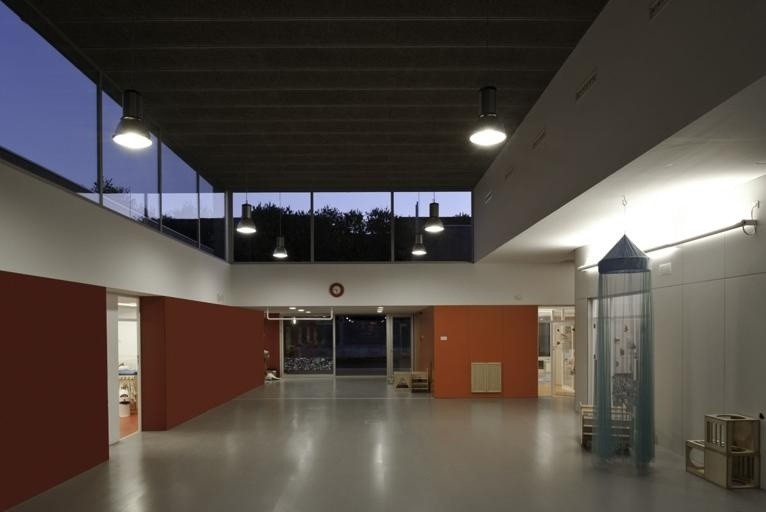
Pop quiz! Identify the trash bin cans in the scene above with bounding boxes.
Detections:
[119,389,130,417]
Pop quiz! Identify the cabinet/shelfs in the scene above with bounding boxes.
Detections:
[684,414,760,490]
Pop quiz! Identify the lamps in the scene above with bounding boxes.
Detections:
[409,190,426,256]
[272,190,289,259]
[111,0,153,152]
[422,190,448,237]
[466,1,507,147]
[234,172,262,236]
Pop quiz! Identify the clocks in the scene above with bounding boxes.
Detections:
[328,283,344,298]
